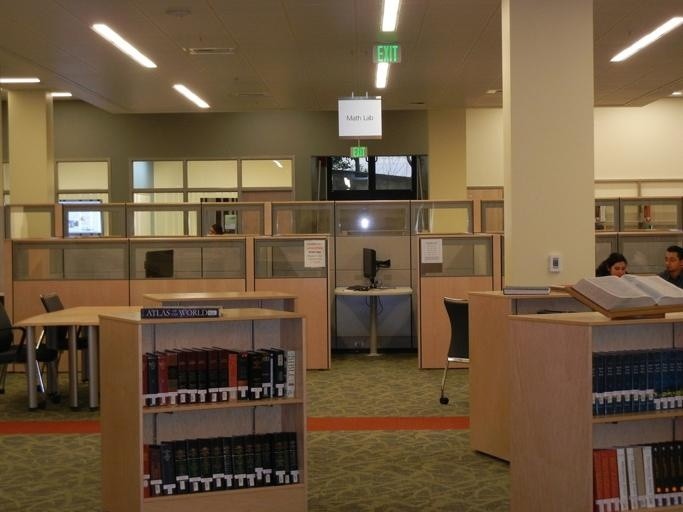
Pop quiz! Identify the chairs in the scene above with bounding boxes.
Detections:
[437,295,470,407]
[0,295,60,405]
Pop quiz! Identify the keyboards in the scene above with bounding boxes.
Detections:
[351,286,366,290]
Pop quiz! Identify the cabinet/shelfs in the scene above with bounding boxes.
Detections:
[98,292,310,510]
[416,177,683,370]
[467,286,681,512]
[2,202,330,376]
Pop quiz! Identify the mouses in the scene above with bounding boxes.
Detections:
[359,287,368,291]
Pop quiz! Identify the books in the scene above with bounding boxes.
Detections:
[502,284,551,295]
[568,272,682,311]
[592,345,682,512]
[139,344,300,499]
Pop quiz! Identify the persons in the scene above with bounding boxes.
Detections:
[655,245,682,289]
[593,251,631,277]
[208,223,223,235]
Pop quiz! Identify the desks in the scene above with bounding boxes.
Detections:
[9,306,142,414]
[335,285,413,359]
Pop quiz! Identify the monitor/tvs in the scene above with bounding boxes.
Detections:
[364,248,376,277]
